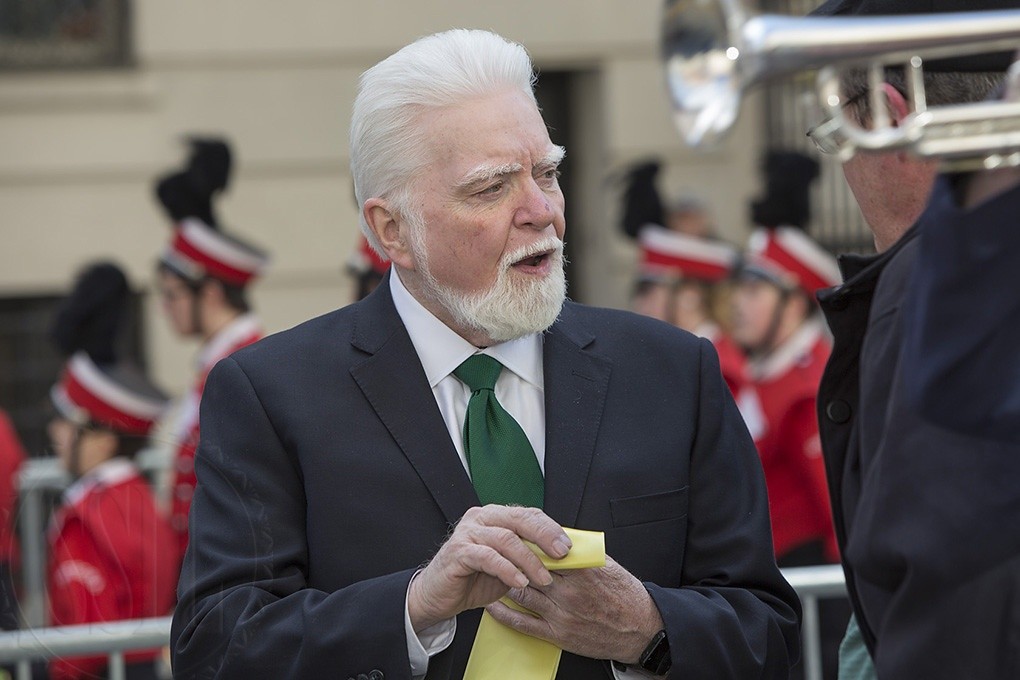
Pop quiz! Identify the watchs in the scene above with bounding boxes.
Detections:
[612,631,672,677]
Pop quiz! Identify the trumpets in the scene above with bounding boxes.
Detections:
[660,0,1020,176]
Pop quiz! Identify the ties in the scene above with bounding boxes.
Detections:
[451,354,544,514]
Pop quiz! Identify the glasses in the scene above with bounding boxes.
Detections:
[806,89,869,156]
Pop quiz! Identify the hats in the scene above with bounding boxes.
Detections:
[45,263,166,438]
[618,166,732,286]
[747,152,839,298]
[159,143,261,290]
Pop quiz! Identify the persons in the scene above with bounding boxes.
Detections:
[45,261,181,680]
[612,158,749,397]
[817,0,1019,679]
[168,26,803,680]
[722,141,855,680]
[146,131,269,546]
